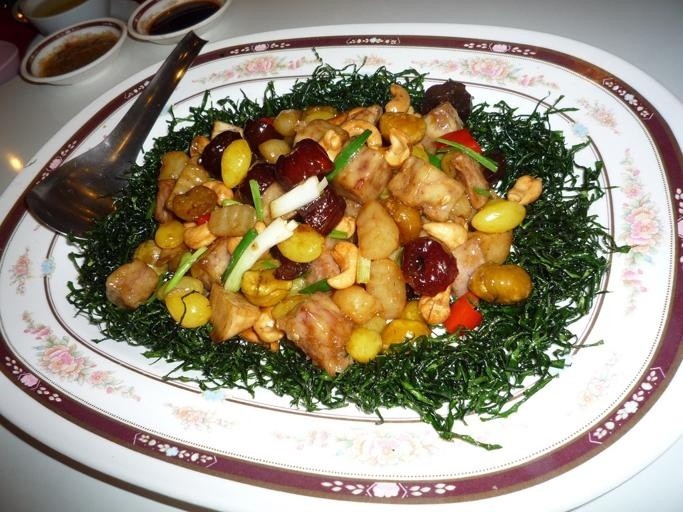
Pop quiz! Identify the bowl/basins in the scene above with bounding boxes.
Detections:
[0,40,20,86]
[19,0,111,39]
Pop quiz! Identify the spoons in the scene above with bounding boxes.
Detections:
[20,29,210,241]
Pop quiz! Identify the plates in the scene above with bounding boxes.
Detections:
[18,15,129,88]
[1,20,683,511]
[127,1,234,46]
